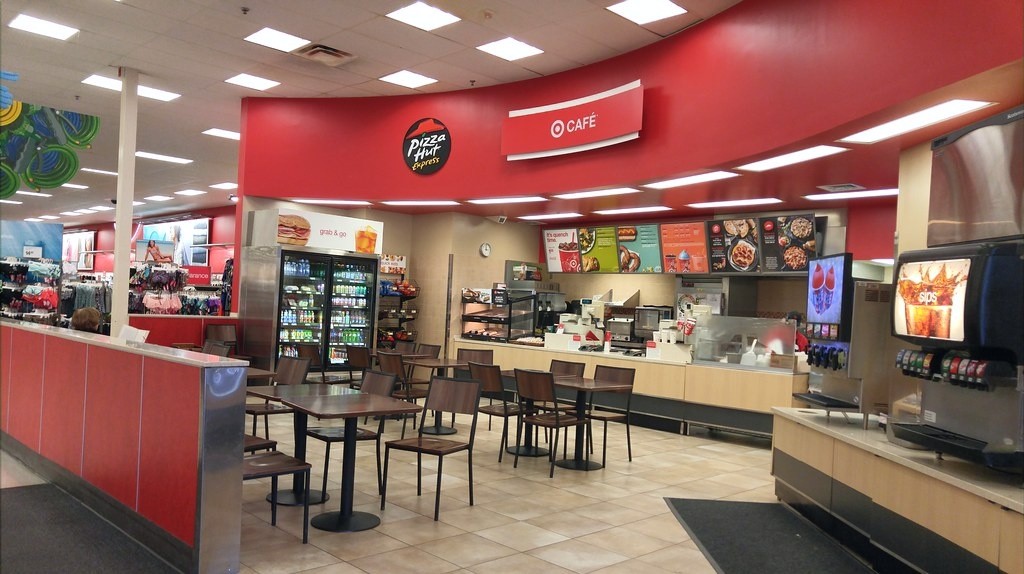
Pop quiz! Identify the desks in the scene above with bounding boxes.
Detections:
[555,377,634,472]
[282,393,424,531]
[247,367,279,378]
[247,384,369,506]
[234,355,253,385]
[496,369,577,457]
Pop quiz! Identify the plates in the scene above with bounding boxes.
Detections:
[728,239,759,272]
[578,229,595,254]
[790,217,814,240]
[783,244,809,270]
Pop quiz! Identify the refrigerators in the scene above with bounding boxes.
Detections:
[236,246,381,372]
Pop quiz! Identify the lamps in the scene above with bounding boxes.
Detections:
[228,194,239,202]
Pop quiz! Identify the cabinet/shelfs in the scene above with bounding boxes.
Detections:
[460,294,537,342]
[376,253,421,365]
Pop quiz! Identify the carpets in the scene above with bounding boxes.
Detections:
[663,495,878,574]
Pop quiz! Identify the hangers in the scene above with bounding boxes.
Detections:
[0,256,220,322]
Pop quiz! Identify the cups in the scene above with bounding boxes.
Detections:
[693,256,706,272]
[931,305,951,338]
[906,304,931,338]
[812,278,825,314]
[679,258,690,272]
[355,230,377,254]
[558,247,581,272]
[665,257,677,272]
[824,284,833,306]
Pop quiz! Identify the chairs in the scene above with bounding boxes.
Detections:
[206,322,256,386]
[521,359,585,443]
[203,339,225,353]
[451,348,493,431]
[407,342,441,418]
[210,344,232,357]
[243,434,278,454]
[394,340,418,389]
[244,450,313,545]
[513,367,590,479]
[376,350,429,429]
[381,375,483,521]
[346,344,400,425]
[563,365,636,468]
[467,361,539,462]
[174,343,204,352]
[297,342,355,421]
[306,369,400,504]
[245,355,313,455]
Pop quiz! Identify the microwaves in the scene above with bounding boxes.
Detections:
[635,306,673,330]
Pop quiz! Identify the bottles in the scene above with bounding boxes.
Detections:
[279,260,367,360]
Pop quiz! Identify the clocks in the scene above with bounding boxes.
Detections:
[480,243,491,257]
